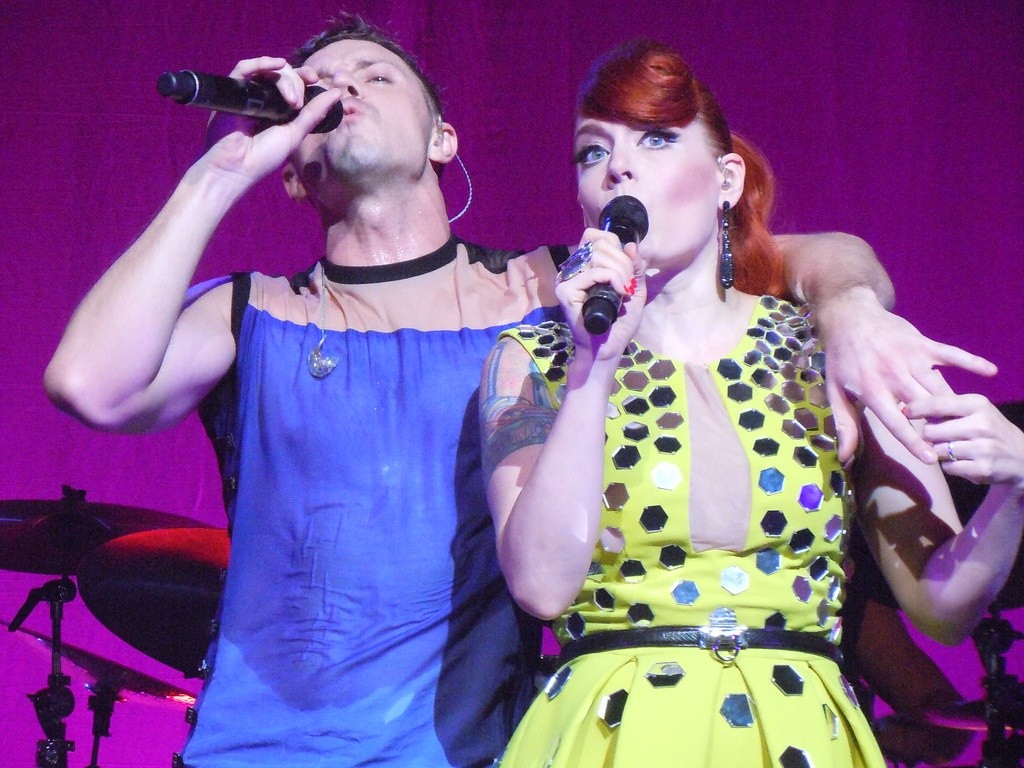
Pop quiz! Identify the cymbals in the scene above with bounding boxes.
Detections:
[0,497,231,708]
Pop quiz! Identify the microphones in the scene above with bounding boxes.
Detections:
[156,69,343,134]
[582,195,648,335]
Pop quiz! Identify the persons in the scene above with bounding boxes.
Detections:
[838,516,977,765]
[40,12,1000,768]
[477,35,1024,768]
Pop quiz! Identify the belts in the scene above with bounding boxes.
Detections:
[557,626,846,675]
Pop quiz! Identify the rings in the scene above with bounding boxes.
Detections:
[558,239,593,283]
[946,440,959,462]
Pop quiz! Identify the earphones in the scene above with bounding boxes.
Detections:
[722,181,731,190]
[306,348,339,378]
